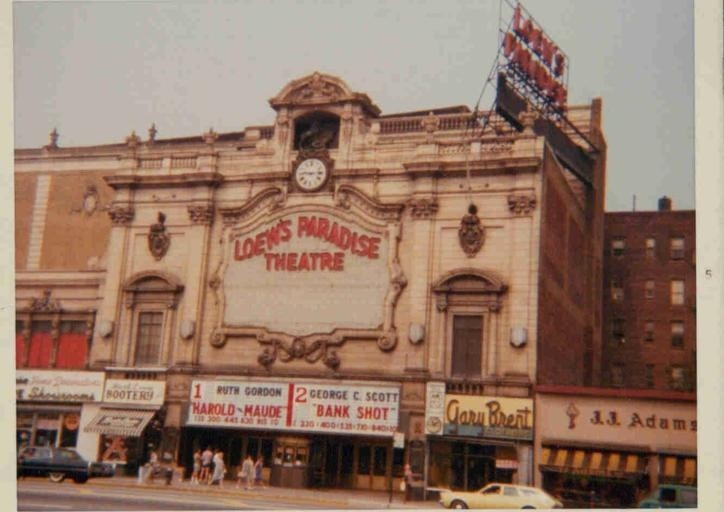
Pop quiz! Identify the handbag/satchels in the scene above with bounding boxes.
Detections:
[237,472,246,478]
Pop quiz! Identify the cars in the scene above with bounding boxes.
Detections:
[437,481,565,510]
[18,446,115,485]
[637,483,698,509]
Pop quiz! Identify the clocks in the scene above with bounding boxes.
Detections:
[295,157,328,192]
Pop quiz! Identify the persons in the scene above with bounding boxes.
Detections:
[253,455,267,490]
[208,448,225,490]
[199,445,213,484]
[142,445,174,485]
[402,463,413,503]
[236,455,254,490]
[190,447,201,486]
